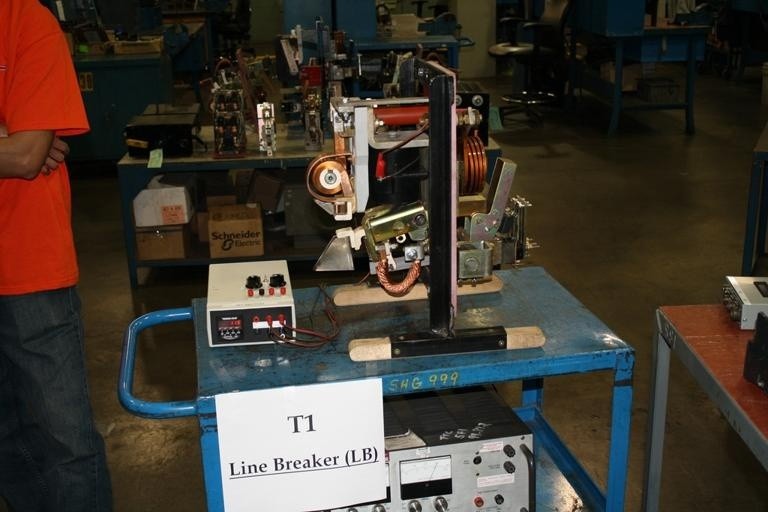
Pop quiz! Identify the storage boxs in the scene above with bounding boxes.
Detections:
[133,169,282,266]
[635,76,680,102]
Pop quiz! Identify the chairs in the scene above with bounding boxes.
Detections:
[487,1,568,126]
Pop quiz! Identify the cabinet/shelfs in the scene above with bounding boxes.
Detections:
[345,36,459,101]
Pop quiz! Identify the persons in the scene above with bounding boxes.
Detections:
[0,0,115,512]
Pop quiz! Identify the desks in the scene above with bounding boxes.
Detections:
[639,293,768,512]
[117,121,503,287]
[572,21,711,143]
